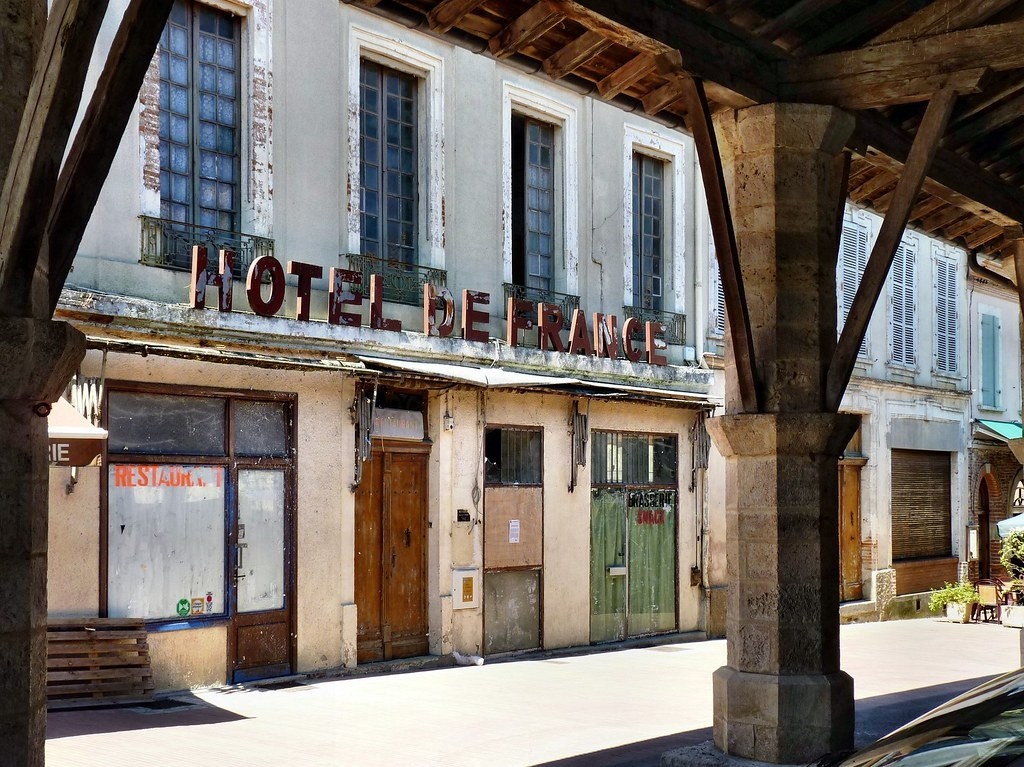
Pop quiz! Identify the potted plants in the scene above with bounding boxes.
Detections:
[997,526,1024,628]
[927,574,985,624]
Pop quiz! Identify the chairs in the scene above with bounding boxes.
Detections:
[973,578,1005,625]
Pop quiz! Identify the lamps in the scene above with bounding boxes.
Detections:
[975,461,992,482]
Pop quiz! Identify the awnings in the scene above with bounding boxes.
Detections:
[976,419,1024,464]
[355,354,725,495]
[64,337,384,497]
[45,394,110,469]
